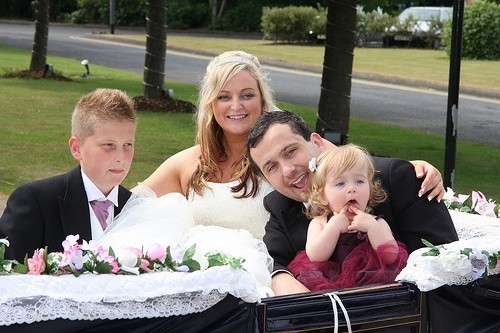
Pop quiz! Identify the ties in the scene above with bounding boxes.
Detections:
[89,199,112,231]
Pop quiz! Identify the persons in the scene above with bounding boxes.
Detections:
[285,143,408,293]
[125,51,444,243]
[0,88,140,268]
[245,110,458,295]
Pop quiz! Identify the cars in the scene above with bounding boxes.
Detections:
[383,7,454,50]
